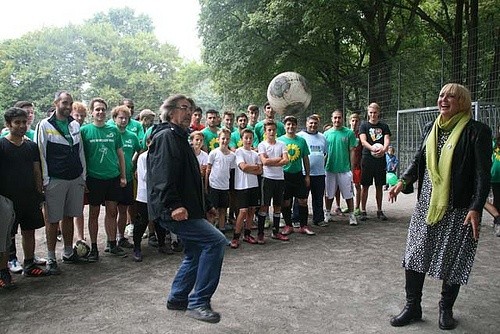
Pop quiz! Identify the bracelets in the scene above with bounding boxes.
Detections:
[399,178,407,191]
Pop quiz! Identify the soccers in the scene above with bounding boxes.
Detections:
[266,72,313,117]
[371,144,384,158]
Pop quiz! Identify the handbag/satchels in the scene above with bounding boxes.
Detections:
[415,167,426,201]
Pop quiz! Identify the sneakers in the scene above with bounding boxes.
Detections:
[21,265,46,277]
[0,272,17,287]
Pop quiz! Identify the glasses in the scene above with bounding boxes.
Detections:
[96,109,105,112]
[174,105,194,112]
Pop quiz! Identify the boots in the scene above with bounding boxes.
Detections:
[437,278,461,330]
[389,269,426,326]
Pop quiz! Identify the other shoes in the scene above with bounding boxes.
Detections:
[57,233,62,242]
[61,240,100,263]
[124,226,148,239]
[158,238,183,255]
[225,239,231,246]
[104,240,110,252]
[230,235,259,249]
[8,260,24,273]
[33,259,47,265]
[167,299,189,311]
[132,247,144,262]
[109,244,128,258]
[255,229,289,245]
[45,257,60,275]
[185,305,221,324]
[147,235,159,247]
[117,237,134,248]
[493,216,500,237]
[264,209,387,236]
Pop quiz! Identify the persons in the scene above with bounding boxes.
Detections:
[0,90,399,323]
[388,83,492,330]
[490,125,500,237]
